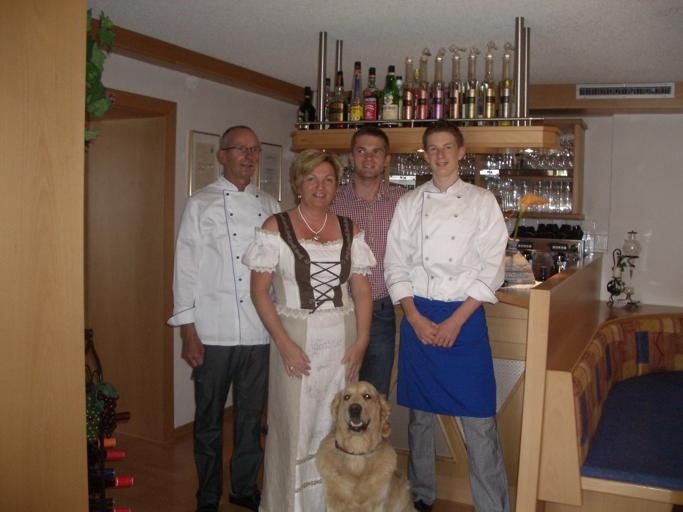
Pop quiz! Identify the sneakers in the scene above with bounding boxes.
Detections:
[228,490,261,512]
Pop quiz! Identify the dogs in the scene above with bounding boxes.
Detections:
[316,380,418,512]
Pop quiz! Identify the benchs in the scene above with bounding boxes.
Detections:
[536,300,683,512]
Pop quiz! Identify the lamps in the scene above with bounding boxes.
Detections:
[607,231,641,309]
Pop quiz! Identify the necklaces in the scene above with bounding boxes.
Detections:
[297,204,329,241]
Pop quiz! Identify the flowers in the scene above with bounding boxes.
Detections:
[514,192,550,238]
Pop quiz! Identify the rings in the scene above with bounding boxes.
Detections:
[289,366,295,371]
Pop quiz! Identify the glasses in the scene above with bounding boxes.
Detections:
[220,147,262,154]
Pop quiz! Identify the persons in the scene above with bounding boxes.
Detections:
[167,125,280,512]
[384,119,509,512]
[241,148,376,512]
[328,127,411,399]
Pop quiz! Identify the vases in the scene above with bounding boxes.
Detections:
[504,235,536,285]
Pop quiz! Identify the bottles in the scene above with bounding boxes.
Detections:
[85,410,135,510]
[297,40,514,125]
[623,230,641,256]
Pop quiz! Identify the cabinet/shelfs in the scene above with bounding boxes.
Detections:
[380,117,587,222]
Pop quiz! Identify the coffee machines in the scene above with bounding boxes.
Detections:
[506,237,582,281]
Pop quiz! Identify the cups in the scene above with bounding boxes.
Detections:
[391,139,573,214]
